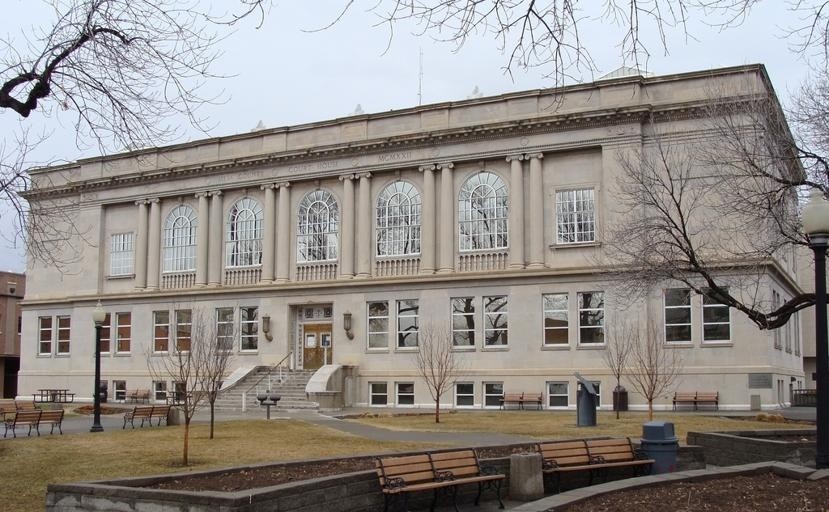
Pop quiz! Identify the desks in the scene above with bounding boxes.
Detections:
[37,388,69,403]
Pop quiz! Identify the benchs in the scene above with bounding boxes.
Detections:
[122,405,170,429]
[370,445,507,511]
[497,391,544,411]
[0,399,64,437]
[534,437,657,492]
[671,391,720,411]
[118,388,150,404]
[32,393,75,403]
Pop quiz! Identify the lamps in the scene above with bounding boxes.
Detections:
[260,312,273,341]
[342,310,355,340]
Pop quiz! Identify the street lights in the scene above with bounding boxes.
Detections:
[802,188,829,469]
[90,298,106,431]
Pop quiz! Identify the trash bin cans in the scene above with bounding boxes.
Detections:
[576,381,599,427]
[751,395,760,410]
[613,386,628,411]
[638,421,679,476]
[167,408,180,426]
[99,385,108,403]
